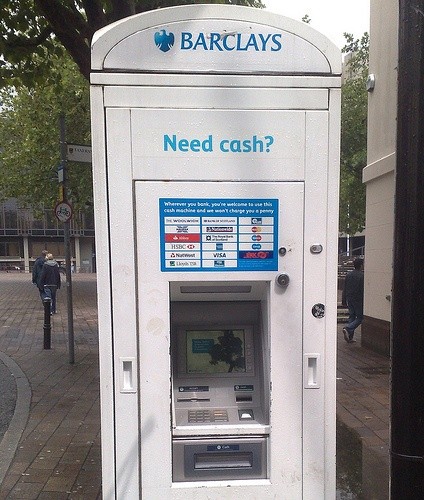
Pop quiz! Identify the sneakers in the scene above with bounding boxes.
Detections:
[342,327,359,343]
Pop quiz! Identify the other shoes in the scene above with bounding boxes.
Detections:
[50,312,53,315]
[54,310,57,314]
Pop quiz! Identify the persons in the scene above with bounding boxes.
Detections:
[342,258,364,343]
[32,250,49,305]
[39,253,61,315]
[61,256,74,276]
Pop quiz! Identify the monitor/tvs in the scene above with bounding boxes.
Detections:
[186,330,246,374]
